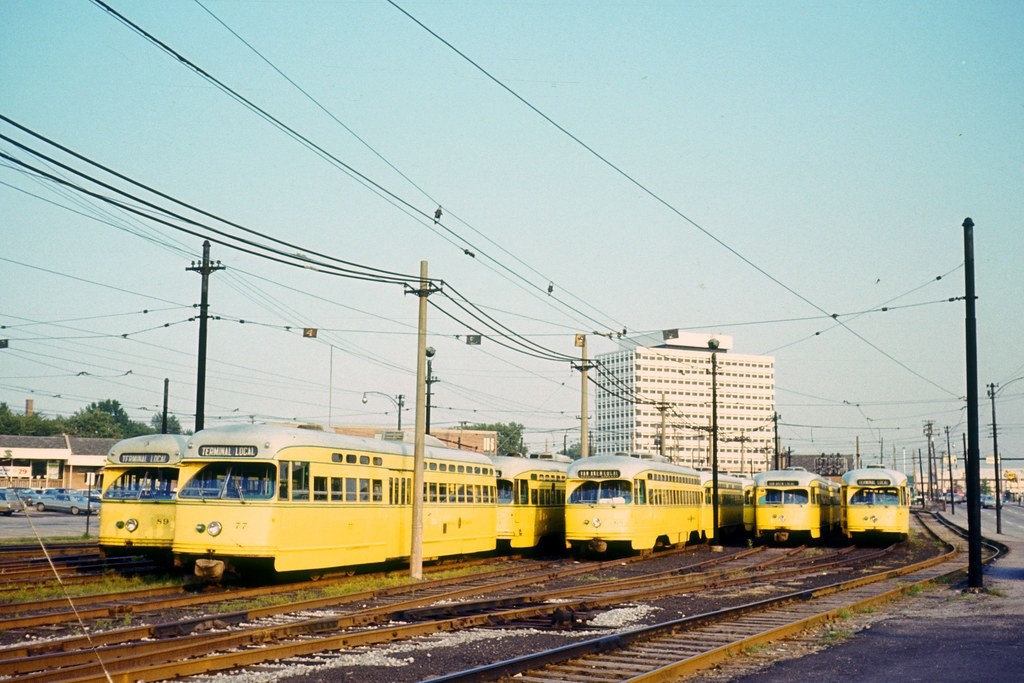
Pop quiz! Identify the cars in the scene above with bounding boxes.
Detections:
[939,492,1004,511]
[0,486,102,516]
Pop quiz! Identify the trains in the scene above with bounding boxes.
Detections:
[564,456,754,555]
[172,424,570,586]
[744,467,843,544]
[840,465,910,540]
[98,435,190,551]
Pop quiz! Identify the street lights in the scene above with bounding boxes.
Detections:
[362,390,405,432]
[943,425,956,515]
